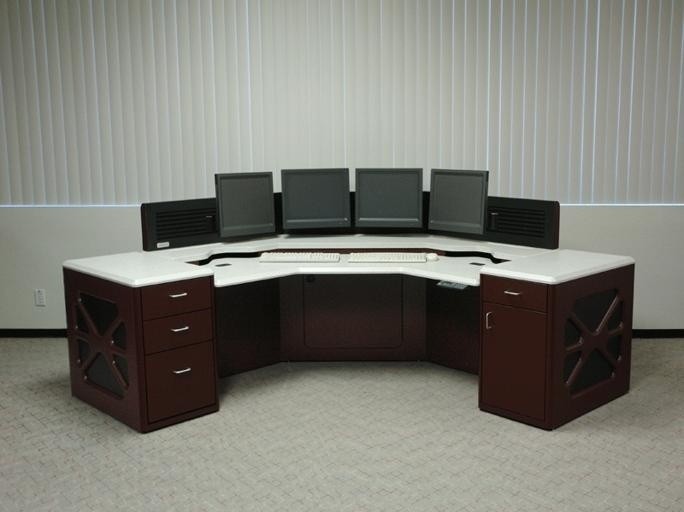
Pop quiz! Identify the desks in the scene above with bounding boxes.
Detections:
[147,232,553,287]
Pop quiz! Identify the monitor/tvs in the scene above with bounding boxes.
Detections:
[355,167,423,228]
[281,167,351,230]
[428,168,489,235]
[214,171,276,237]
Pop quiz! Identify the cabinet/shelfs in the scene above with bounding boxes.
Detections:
[62,234,220,434]
[477,248,636,431]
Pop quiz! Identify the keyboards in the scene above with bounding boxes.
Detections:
[347,251,426,263]
[258,251,341,264]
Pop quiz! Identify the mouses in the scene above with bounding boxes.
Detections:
[426,251,439,262]
